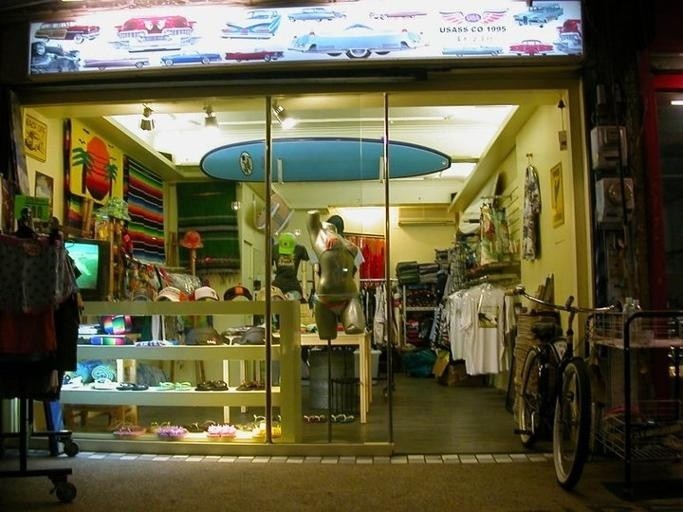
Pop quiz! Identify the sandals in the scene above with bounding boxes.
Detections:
[66,376,267,392]
[111,420,281,442]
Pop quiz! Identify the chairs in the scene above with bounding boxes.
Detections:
[318,319,395,444]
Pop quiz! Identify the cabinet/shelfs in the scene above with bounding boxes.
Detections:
[397,281,442,352]
[58,299,306,443]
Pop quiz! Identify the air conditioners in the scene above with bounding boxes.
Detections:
[394,204,456,226]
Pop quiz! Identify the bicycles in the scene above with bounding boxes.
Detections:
[507,285,627,488]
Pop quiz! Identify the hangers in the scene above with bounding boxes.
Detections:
[0,211,75,252]
[358,279,394,291]
[478,194,506,214]
[451,269,494,291]
[525,153,535,167]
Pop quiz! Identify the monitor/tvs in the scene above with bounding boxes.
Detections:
[62,237,110,299]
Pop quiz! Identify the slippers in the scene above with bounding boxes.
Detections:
[302,411,356,425]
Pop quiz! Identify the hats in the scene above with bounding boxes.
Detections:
[182,324,222,346]
[178,230,205,250]
[256,286,285,304]
[156,286,183,303]
[276,232,297,256]
[239,324,266,344]
[98,195,132,222]
[190,284,220,303]
[222,285,253,301]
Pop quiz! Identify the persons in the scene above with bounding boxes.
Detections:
[304,209,365,341]
[269,233,309,303]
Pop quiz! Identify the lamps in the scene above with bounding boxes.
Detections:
[137,95,297,151]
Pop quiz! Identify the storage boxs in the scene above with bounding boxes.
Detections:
[432,353,488,390]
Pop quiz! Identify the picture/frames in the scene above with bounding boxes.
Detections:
[32,170,53,218]
[548,159,565,230]
[23,112,48,164]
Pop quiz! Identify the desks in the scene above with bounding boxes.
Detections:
[273,330,374,424]
